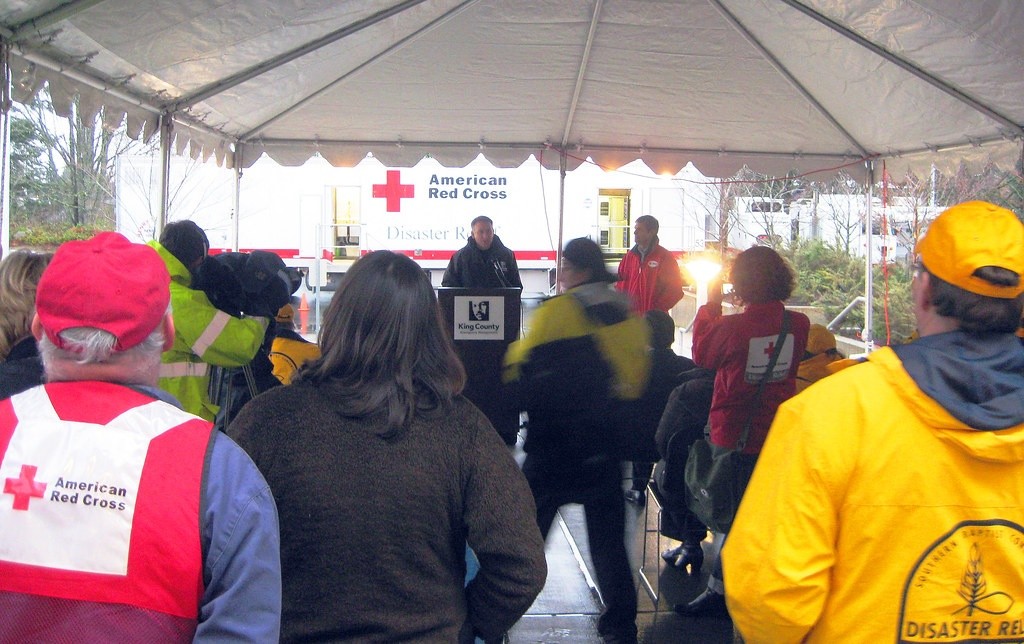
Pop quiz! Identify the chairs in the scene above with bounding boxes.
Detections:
[638,430,702,607]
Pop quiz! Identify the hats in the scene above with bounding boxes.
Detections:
[35,231,171,354]
[562,237,623,281]
[274,303,295,323]
[804,324,836,355]
[915,200,1024,298]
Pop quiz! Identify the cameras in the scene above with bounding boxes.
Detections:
[720,282,736,295]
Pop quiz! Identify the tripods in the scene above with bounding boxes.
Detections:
[208,358,259,431]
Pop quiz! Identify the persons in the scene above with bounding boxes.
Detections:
[0,214,867,618]
[720,200,1024,644]
[501,239,654,644]
[1,233,284,644]
[223,251,547,643]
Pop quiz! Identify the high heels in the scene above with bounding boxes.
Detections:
[661,544,704,576]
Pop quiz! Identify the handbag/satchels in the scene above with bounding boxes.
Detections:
[684,439,744,533]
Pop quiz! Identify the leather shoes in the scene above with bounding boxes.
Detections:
[673,586,729,617]
[624,489,646,506]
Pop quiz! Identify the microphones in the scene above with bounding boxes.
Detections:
[491,258,512,287]
